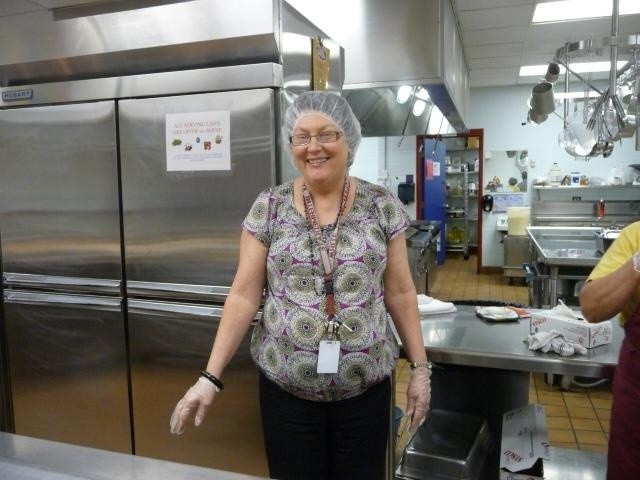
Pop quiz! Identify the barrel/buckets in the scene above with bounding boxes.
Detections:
[507,207,530,236]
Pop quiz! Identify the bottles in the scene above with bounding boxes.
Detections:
[549,162,561,187]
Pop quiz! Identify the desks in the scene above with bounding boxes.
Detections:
[385,304,628,479]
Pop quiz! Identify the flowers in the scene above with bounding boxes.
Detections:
[487,176,503,192]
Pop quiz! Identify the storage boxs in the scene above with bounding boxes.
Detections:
[467,136,479,148]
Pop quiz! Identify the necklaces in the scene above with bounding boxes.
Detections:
[301,190,344,298]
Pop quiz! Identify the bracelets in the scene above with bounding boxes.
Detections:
[199,370,226,390]
[410,361,433,370]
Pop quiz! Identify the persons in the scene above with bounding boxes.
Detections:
[168,90,434,480]
[578,220,640,479]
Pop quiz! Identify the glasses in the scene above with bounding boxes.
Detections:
[288,129,344,147]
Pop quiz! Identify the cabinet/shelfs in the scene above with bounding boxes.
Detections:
[445,147,480,259]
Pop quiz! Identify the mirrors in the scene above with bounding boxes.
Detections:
[484,150,528,193]
